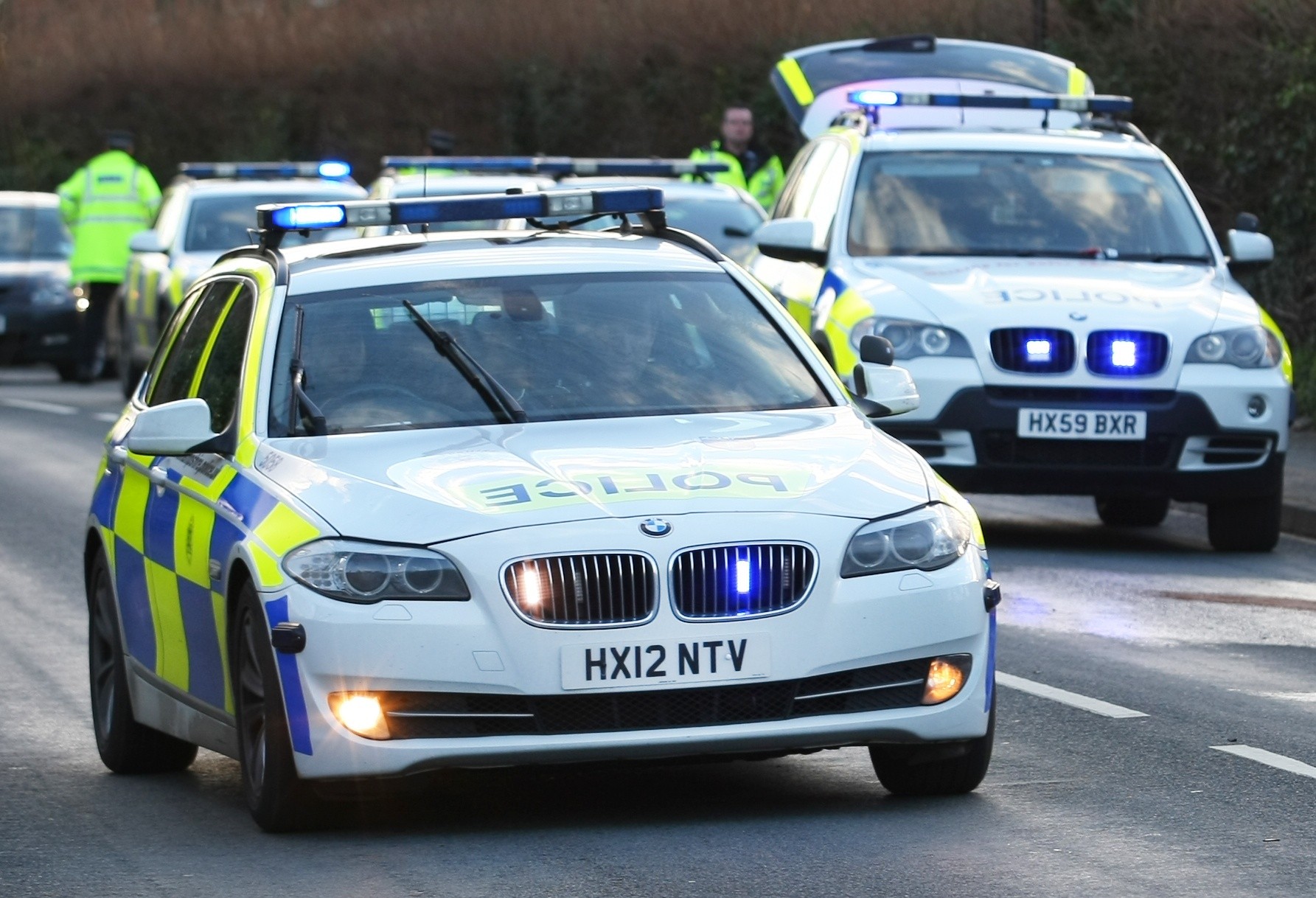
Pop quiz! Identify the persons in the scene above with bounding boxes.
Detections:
[680,104,786,210]
[295,291,677,407]
[56,131,163,384]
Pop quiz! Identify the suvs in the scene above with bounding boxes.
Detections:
[718,32,1296,549]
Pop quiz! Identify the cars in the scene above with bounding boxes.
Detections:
[104,152,771,402]
[82,187,1005,833]
[0,189,86,373]
